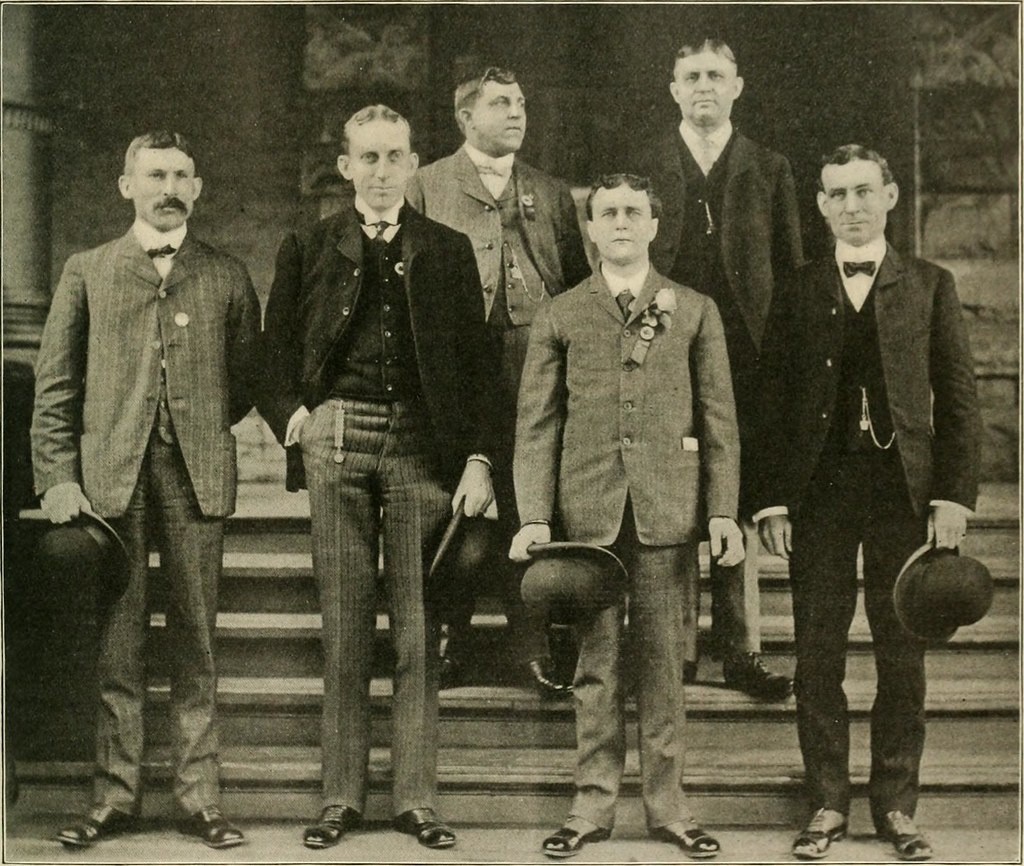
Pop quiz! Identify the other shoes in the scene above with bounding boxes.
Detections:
[432,656,455,688]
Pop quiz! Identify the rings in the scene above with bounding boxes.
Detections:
[958,533,966,539]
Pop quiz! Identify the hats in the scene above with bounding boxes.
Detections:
[892,541,994,642]
[428,496,493,583]
[521,542,629,615]
[32,508,131,611]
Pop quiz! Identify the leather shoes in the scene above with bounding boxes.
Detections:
[518,656,573,699]
[542,816,611,858]
[874,810,933,860]
[175,803,244,848]
[648,818,720,858]
[394,807,455,848]
[723,652,794,700]
[304,805,362,848]
[791,808,849,859]
[55,803,134,846]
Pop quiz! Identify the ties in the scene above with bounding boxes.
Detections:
[615,289,635,323]
[354,204,405,246]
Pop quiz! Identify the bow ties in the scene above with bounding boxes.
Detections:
[843,261,876,277]
[147,244,177,259]
[476,165,503,177]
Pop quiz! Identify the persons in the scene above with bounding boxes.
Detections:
[510,173,746,858]
[407,66,595,696]
[626,36,797,701]
[751,144,983,860]
[31,129,263,849]
[256,105,508,849]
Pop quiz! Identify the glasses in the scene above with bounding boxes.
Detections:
[478,67,524,84]
[592,173,649,191]
